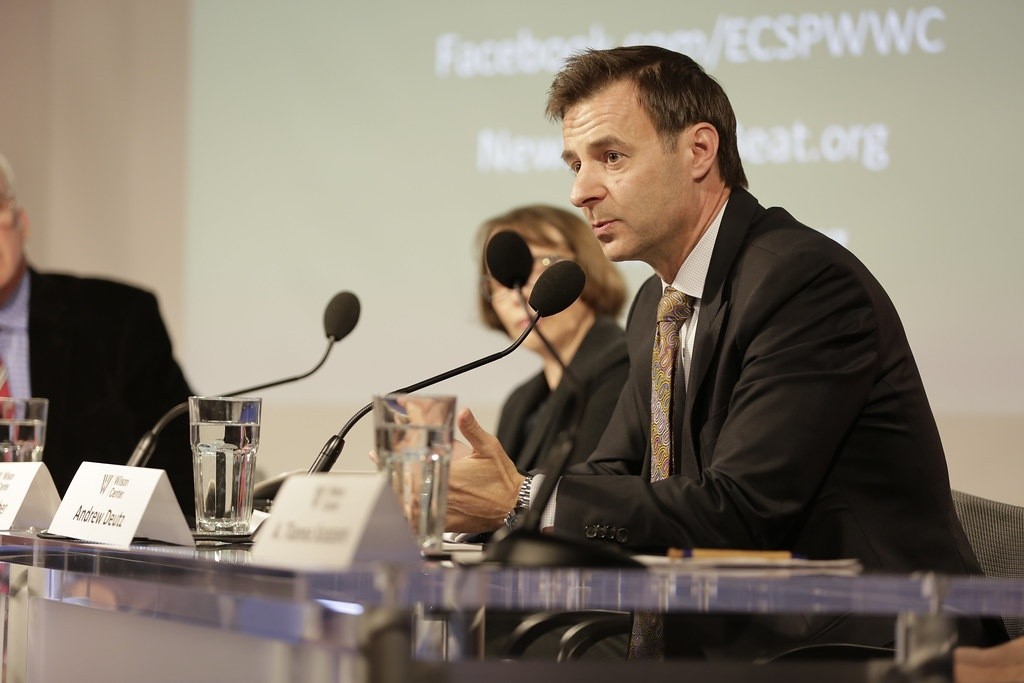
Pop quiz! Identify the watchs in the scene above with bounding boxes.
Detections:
[504,477,534,530]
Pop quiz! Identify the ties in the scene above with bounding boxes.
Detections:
[626,285,696,662]
[0,358,13,424]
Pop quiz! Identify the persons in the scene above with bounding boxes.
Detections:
[477,204,628,471]
[367,45,1009,660]
[0,167,238,528]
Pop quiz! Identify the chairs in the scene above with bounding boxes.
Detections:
[495,489,1024,662]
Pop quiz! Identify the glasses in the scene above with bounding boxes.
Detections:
[481,254,565,302]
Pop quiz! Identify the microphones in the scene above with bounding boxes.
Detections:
[308,230,650,579]
[129,292,361,470]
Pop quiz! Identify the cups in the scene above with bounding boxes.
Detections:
[373,395,456,568]
[188,397,262,546]
[0,398,49,462]
